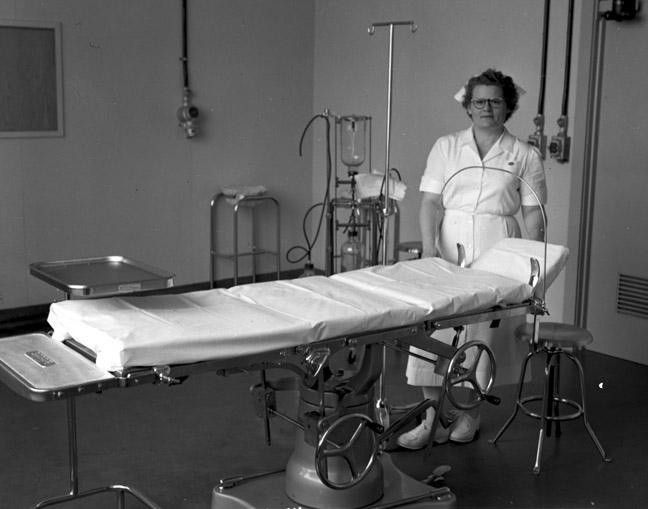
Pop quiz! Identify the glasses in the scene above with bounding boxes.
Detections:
[471,98,504,109]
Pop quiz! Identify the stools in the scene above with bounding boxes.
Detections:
[487,322,614,476]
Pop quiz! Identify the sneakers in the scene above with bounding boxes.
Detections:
[398,420,449,450]
[448,411,480,442]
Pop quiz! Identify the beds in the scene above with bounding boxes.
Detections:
[0,235,571,508]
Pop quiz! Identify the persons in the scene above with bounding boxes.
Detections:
[395,68,548,450]
